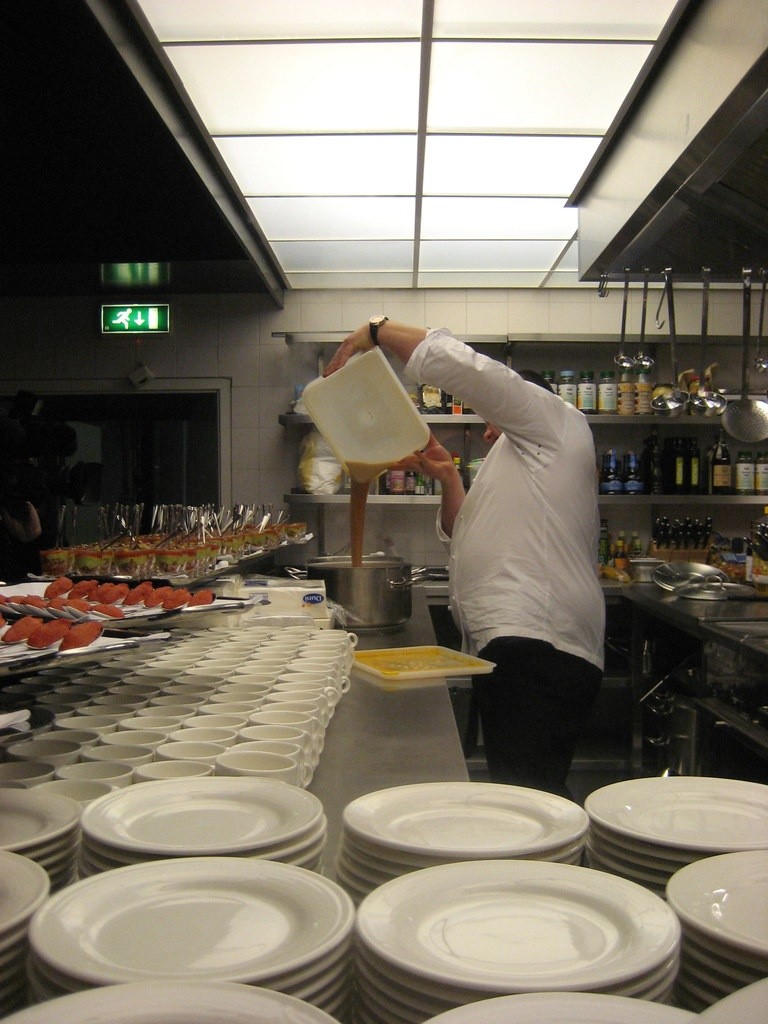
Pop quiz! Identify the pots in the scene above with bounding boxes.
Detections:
[284,555,436,631]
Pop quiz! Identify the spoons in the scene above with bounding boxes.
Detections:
[0,593,263,664]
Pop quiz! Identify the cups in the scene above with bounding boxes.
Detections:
[40,523,309,581]
[0,625,359,810]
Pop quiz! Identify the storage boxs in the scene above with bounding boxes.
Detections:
[300,346,431,485]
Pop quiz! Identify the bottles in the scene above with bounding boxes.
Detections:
[597,519,642,577]
[599,430,700,495]
[705,428,768,495]
[408,384,476,414]
[653,384,674,416]
[540,366,652,415]
[379,457,464,495]
[751,506,768,598]
[687,376,712,416]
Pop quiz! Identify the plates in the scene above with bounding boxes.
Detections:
[0,777,768,1024]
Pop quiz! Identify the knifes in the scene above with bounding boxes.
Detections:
[651,517,713,550]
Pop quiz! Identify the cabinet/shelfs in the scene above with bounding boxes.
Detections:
[273,332,767,604]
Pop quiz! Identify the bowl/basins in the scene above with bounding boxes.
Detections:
[690,389,727,415]
[650,391,690,416]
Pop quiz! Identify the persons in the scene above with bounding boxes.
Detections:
[0,399,61,584]
[323,316,607,802]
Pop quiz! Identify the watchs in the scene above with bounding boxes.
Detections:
[368,317,387,347]
